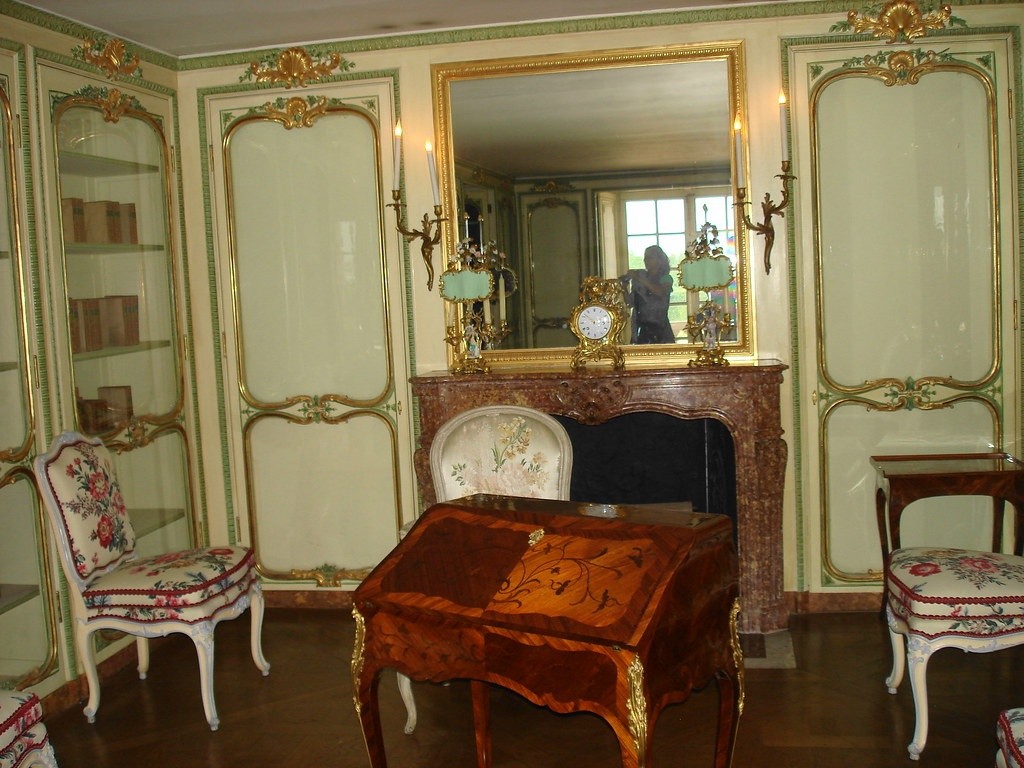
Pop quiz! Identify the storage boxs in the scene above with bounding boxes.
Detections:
[78,386,133,435]
[67,295,139,354]
[61,198,140,245]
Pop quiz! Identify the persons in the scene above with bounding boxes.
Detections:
[622,246,676,345]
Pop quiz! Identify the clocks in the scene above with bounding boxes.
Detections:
[568,275,630,372]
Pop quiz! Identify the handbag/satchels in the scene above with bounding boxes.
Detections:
[637,298,665,327]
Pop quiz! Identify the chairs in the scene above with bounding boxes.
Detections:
[0,687,59,768]
[398,404,573,733]
[33,431,271,731]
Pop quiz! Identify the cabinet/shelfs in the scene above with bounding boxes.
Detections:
[30,39,203,655]
[0,37,64,694]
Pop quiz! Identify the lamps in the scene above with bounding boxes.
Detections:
[385,119,450,292]
[733,88,796,275]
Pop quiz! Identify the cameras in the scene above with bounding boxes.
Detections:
[622,274,631,282]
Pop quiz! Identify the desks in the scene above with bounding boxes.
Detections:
[868,452,1024,622]
[349,493,746,768]
[407,357,790,634]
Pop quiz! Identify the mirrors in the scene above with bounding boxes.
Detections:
[430,39,754,370]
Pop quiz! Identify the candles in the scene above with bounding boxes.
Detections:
[724,286,729,315]
[687,290,695,317]
[482,300,492,325]
[448,301,453,326]
[499,271,506,321]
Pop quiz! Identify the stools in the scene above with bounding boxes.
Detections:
[995,707,1024,768]
[885,547,1024,760]
